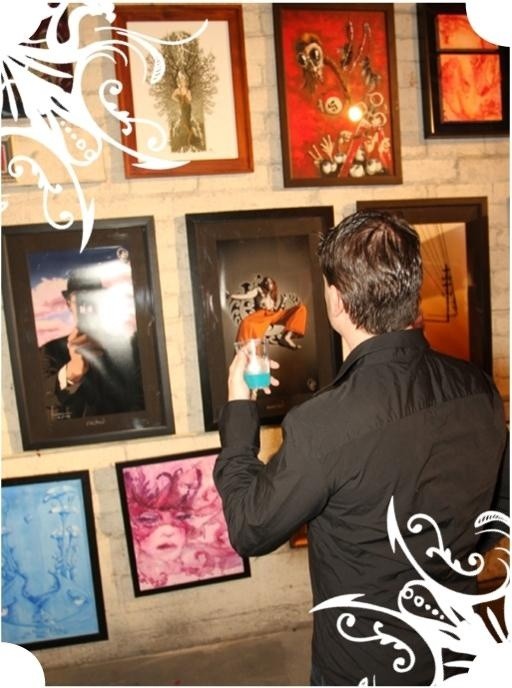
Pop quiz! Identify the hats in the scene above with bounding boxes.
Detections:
[63,274,107,295]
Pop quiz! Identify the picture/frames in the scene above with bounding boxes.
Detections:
[1,3,508,656]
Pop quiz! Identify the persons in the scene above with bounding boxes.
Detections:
[42,257,140,427]
[213,206,509,685]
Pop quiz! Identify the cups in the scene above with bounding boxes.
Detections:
[232,335,273,390]
